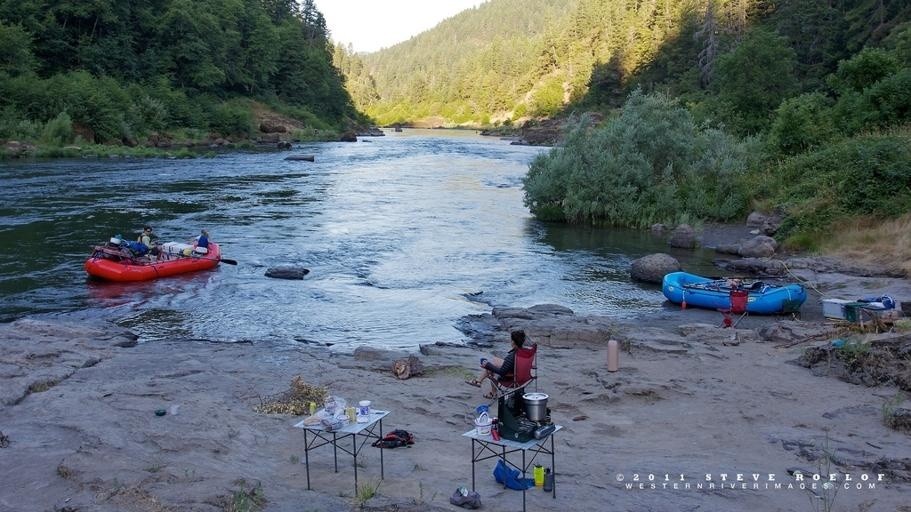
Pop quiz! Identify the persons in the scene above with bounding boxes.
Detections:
[715,311,732,329]
[465,331,526,400]
[137,226,158,262]
[193,229,209,248]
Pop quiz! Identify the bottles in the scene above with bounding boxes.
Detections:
[491,428,501,440]
[682,301,687,308]
[543,468,553,490]
[534,462,545,486]
[491,418,499,432]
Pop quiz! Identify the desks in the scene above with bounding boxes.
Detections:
[292,407,390,494]
[460,416,564,511]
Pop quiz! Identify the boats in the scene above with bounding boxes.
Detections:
[81,240,222,285]
[658,268,806,315]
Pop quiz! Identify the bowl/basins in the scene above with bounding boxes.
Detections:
[338,415,351,426]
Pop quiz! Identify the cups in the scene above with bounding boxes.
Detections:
[359,400,372,416]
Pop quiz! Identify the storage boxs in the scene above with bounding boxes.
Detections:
[820,297,847,318]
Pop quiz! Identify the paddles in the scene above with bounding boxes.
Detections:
[702,275,787,280]
[161,249,238,266]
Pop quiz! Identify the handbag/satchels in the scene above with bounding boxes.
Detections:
[450,487,481,509]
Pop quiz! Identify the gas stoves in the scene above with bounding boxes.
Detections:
[509,412,556,440]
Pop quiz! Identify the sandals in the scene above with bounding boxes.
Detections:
[464,379,480,387]
[482,392,496,400]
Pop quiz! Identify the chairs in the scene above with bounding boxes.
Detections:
[485,345,539,411]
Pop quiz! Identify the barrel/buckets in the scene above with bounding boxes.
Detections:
[475,411,493,435]
